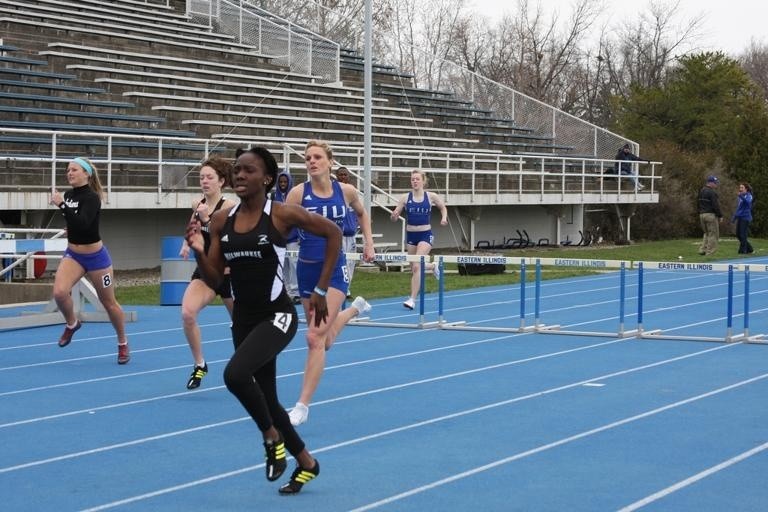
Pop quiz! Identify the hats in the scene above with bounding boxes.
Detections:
[707,176,720,185]
[623,144,630,150]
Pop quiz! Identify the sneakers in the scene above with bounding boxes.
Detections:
[288,401,309,426]
[187,361,208,389]
[118,342,130,364]
[403,298,415,310]
[263,430,287,481]
[432,262,439,281]
[352,295,371,314]
[279,458,320,495]
[59,319,81,347]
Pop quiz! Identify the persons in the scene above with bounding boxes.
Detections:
[336,166,374,300]
[283,139,376,427]
[390,169,449,310]
[183,146,345,495]
[49,156,131,365]
[179,156,246,389]
[271,170,302,306]
[726,181,757,255]
[614,144,649,191]
[698,175,724,258]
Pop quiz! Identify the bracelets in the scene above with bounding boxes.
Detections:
[312,284,330,298]
[202,217,212,226]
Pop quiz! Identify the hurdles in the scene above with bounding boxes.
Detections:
[343,253,447,329]
[632,259,745,342]
[530,257,644,338]
[432,253,545,332]
[286,251,371,324]
[736,263,768,344]
